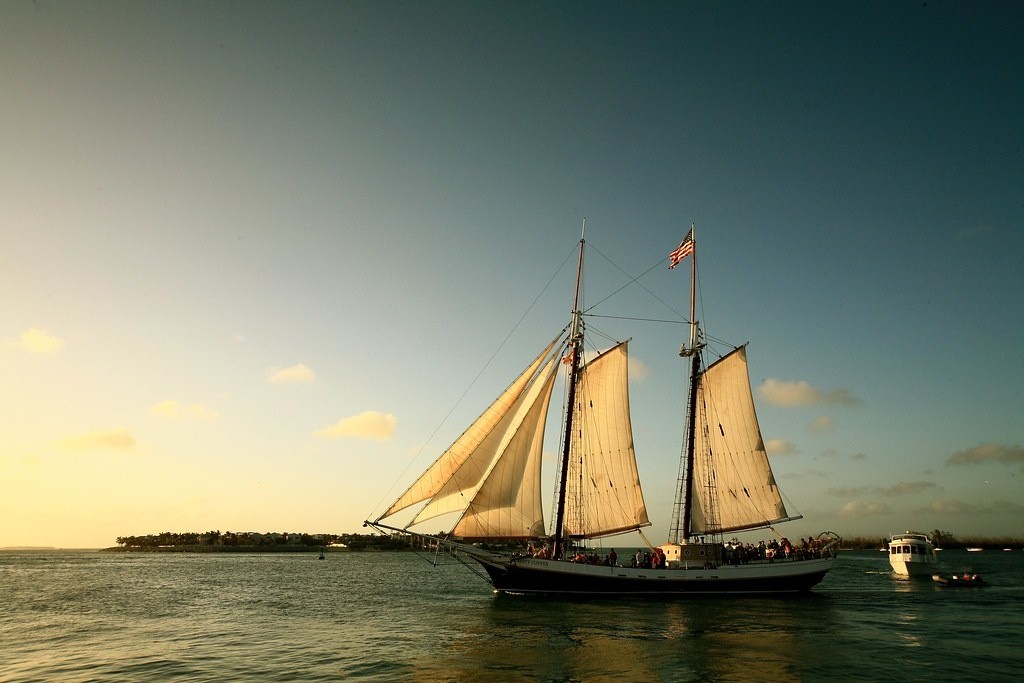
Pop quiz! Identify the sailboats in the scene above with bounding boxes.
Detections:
[361,216,844,595]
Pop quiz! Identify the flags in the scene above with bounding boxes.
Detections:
[668,226,694,269]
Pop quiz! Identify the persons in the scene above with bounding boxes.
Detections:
[526,541,667,570]
[952,572,979,581]
[721,537,838,565]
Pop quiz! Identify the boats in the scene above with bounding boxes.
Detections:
[933,574,990,587]
[886,531,938,576]
